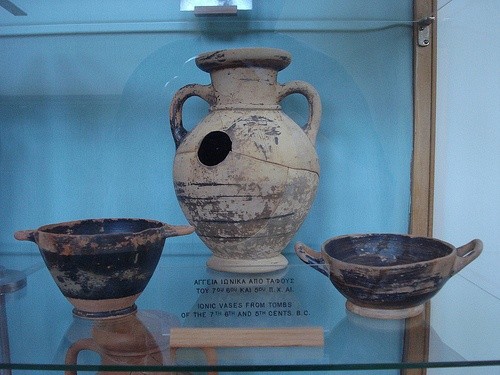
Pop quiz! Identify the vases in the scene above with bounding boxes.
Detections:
[170,49,322,273]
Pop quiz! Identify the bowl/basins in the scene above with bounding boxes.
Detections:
[293,232,486,318]
[11,218,202,320]
